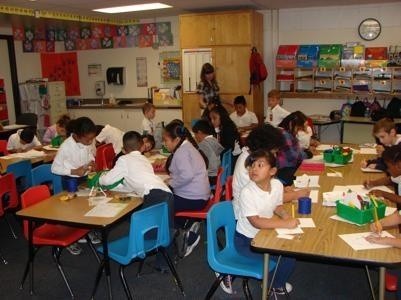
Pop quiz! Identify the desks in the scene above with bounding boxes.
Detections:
[13,148,171,299]
[251,143,400,299]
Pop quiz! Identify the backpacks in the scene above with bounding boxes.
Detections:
[250,47,267,85]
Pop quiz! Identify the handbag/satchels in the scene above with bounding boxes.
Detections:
[341,102,381,117]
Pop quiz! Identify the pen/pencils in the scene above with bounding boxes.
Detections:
[327,167,344,180]
[371,194,378,207]
[292,204,295,219]
[375,208,381,238]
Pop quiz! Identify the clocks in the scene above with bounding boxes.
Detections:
[357,18,381,41]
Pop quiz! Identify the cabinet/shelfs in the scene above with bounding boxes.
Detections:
[275,66,401,106]
[178,11,263,47]
[179,49,264,132]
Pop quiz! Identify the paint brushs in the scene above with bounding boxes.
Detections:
[307,179,310,189]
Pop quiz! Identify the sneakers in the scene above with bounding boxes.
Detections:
[65,231,102,255]
[260,282,294,300]
[174,219,201,259]
[215,271,234,295]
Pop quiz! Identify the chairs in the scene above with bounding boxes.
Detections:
[95,142,111,170]
[222,175,233,199]
[17,185,105,298]
[208,149,232,190]
[101,146,117,169]
[202,202,291,299]
[90,201,186,299]
[0,139,57,238]
[174,166,224,254]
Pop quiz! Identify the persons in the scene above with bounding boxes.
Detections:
[6,114,70,154]
[162,97,242,258]
[361,117,400,291]
[195,63,235,117]
[214,90,320,296]
[95,131,175,279]
[50,117,101,255]
[96,101,162,157]
